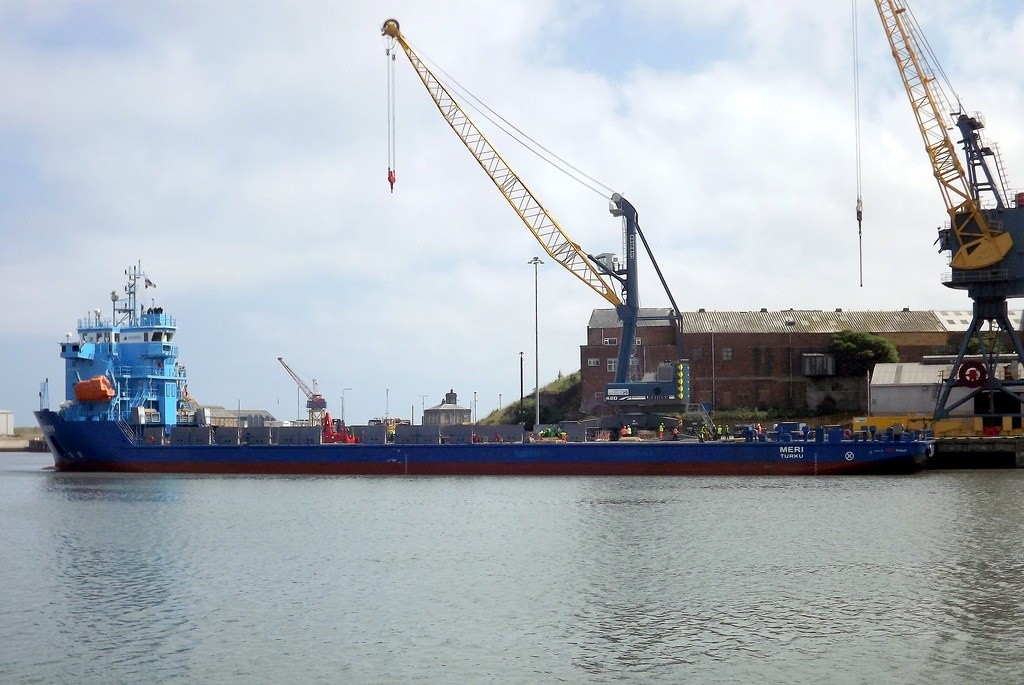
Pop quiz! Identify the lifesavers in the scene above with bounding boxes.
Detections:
[843,428,852,437]
[147,435,155,443]
[958,361,986,388]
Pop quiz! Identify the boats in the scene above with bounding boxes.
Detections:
[34,260,936,473]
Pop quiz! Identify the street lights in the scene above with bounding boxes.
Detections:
[527,257,545,426]
[342,388,353,430]
[518,351,525,423]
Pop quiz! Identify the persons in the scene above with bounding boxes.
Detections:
[620,425,627,437]
[388,427,395,441]
[716,425,723,439]
[725,425,730,439]
[659,423,665,438]
[701,424,707,441]
[756,423,761,433]
[626,425,631,437]
[673,426,680,440]
[561,431,567,443]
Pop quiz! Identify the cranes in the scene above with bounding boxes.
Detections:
[378,19,695,430]
[278,356,358,442]
[850,0,1024,421]
